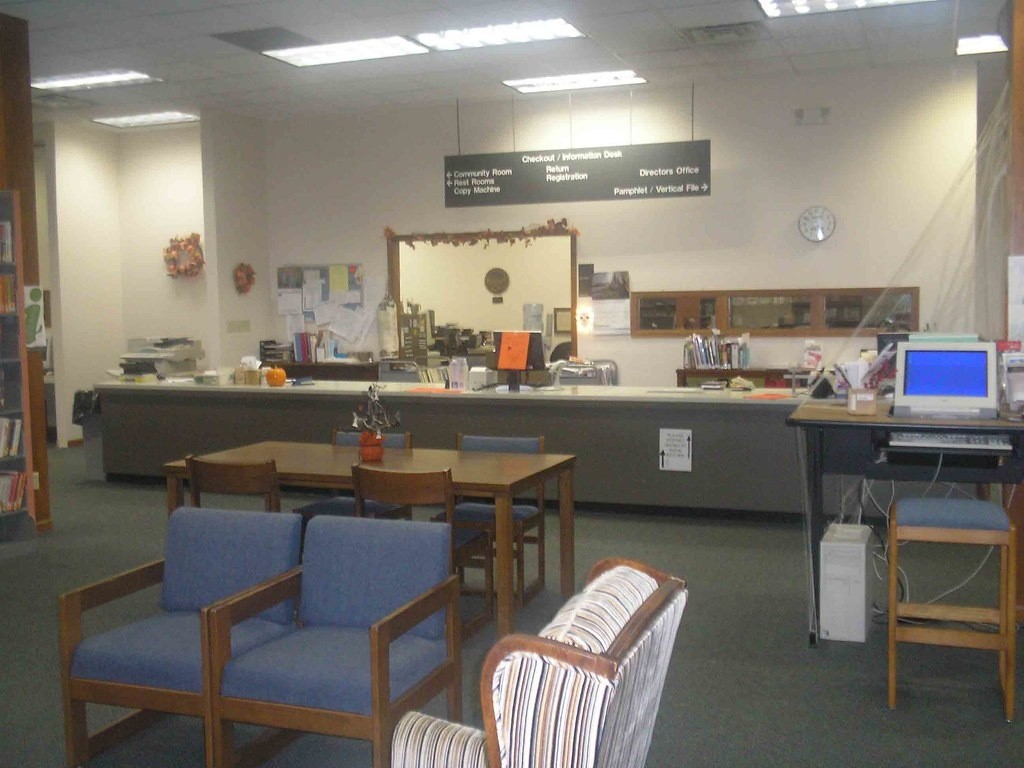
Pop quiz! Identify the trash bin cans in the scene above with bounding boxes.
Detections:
[75,389,108,482]
[559,360,617,386]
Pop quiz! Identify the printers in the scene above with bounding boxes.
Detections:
[118,337,206,379]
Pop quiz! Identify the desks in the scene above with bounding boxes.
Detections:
[265,362,379,380]
[787,397,1024,662]
[162,440,577,639]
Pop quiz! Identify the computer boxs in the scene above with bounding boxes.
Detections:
[819,524,875,643]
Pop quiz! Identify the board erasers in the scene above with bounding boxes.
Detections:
[699,381,727,390]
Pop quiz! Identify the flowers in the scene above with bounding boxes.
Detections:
[164,233,206,279]
[234,262,255,293]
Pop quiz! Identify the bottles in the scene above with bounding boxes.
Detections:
[449,359,467,390]
[1006,366,1024,421]
[523,304,544,330]
[996,340,1021,417]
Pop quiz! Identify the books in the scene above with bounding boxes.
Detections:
[263,330,357,365]
[685,333,748,370]
[700,376,753,392]
[0,220,26,517]
[418,367,450,384]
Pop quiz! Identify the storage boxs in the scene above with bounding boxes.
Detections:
[246,369,262,384]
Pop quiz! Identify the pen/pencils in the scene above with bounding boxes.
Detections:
[834,364,852,388]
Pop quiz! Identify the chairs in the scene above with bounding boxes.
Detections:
[352,463,495,641]
[290,428,414,520]
[391,556,688,768]
[61,506,302,768]
[429,433,544,609]
[208,516,464,768]
[184,455,280,514]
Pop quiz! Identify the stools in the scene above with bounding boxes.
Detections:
[888,498,1020,724]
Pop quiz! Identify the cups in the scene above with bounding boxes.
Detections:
[245,370,260,385]
[194,375,203,384]
[848,388,878,415]
[266,369,286,386]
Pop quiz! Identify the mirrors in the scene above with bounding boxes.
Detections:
[388,230,578,363]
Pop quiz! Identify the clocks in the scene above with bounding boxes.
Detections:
[798,206,836,243]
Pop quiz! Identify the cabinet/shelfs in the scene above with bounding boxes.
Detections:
[0,13,54,534]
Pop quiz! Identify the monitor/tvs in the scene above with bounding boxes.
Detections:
[894,341,998,421]
[494,332,547,394]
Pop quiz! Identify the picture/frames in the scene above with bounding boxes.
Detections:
[553,308,571,333]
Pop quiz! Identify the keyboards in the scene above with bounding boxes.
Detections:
[888,431,1013,451]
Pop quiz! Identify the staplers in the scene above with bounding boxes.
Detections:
[292,376,315,387]
